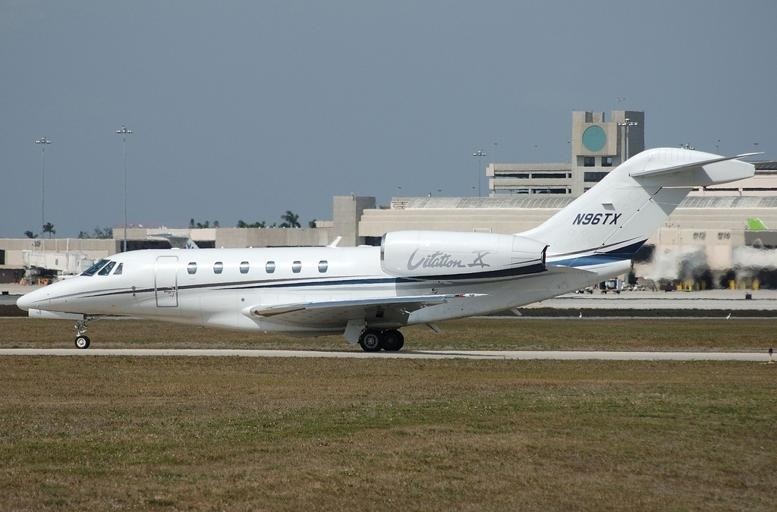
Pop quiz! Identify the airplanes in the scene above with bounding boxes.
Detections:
[14,146,764,353]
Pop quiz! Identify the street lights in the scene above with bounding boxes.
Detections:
[115,121,135,252]
[471,148,486,196]
[33,137,53,235]
